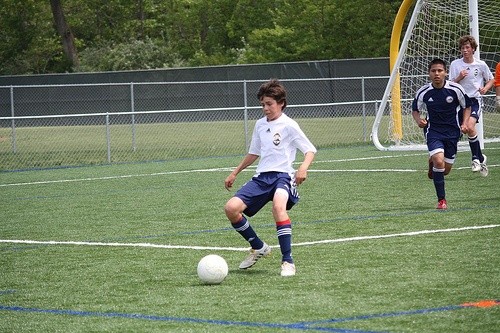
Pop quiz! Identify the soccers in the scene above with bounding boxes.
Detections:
[196,254,229,285]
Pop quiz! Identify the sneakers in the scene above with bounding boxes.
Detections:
[239,242,271,269]
[471,159,481,172]
[480,154,488,177]
[428,158,434,179]
[280,261,296,276]
[437,199,447,209]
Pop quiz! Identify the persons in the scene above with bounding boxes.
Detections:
[448,35,495,177]
[493,62,500,112]
[224,78,317,277]
[412,59,472,209]
